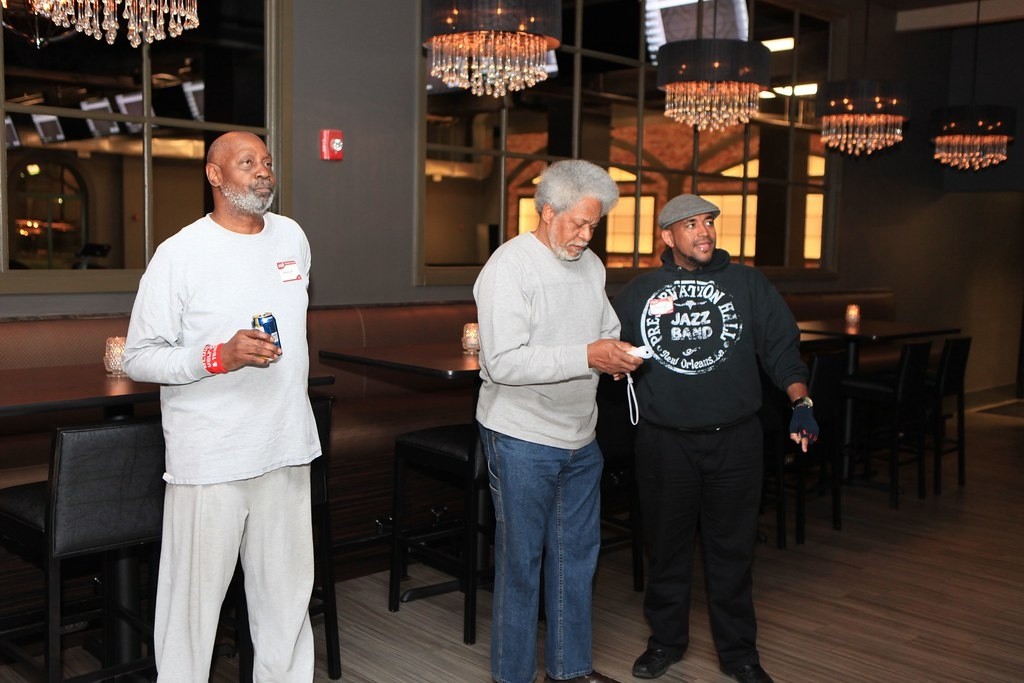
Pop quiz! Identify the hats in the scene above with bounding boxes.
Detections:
[658,193,722,230]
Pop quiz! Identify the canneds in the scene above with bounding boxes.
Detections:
[251,312,283,361]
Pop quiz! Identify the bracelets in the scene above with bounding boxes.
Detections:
[202,343,229,374]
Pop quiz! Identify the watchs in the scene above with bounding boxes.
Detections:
[792,396,814,410]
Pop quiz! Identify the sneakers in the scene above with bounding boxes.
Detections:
[631,643,679,677]
[720,659,772,683]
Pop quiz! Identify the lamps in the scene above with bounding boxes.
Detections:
[929,0,1017,170]
[30,0,200,48]
[421,0,562,98]
[658,0,770,131]
[816,0,909,157]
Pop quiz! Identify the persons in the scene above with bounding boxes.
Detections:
[124,130,323,683]
[472,158,643,683]
[612,192,821,683]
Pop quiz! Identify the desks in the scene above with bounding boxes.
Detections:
[1,364,335,683]
[796,316,962,495]
[757,328,845,545]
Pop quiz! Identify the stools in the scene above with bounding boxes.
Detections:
[760,337,971,550]
[212,396,341,683]
[1,417,165,683]
[375,377,546,645]
[594,373,643,592]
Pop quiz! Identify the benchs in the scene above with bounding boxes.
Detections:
[0,302,545,621]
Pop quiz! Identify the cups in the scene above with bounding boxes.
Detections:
[103,336,128,378]
[461,322,479,355]
[845,304,859,325]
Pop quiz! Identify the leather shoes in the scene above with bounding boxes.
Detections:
[544,668,619,683]
